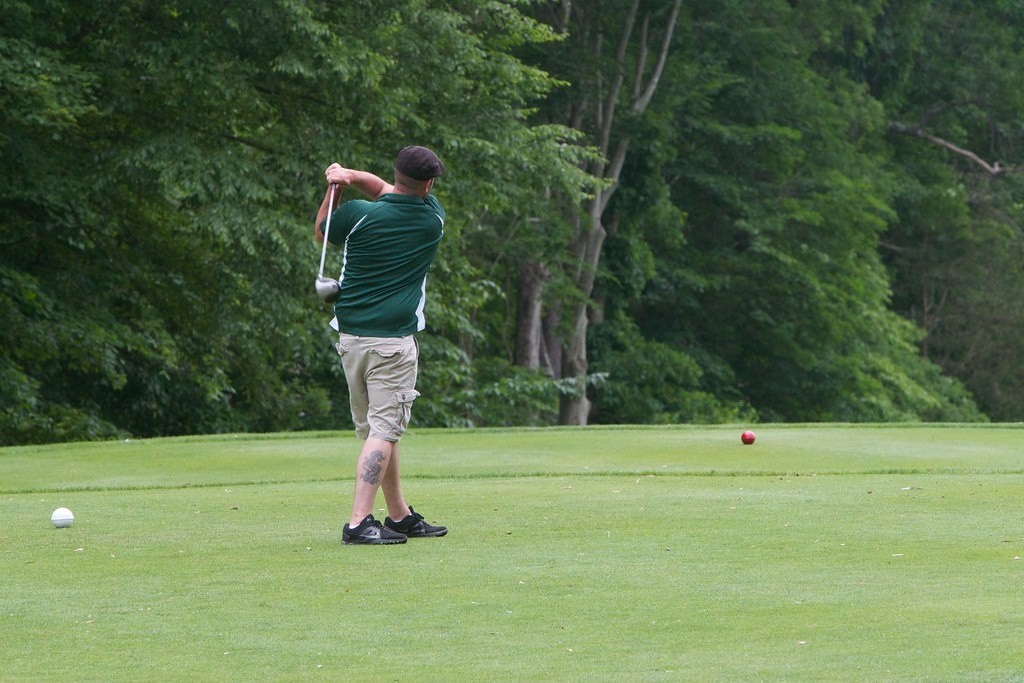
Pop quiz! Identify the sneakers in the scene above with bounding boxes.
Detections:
[341,514,407,544]
[384,505,448,537]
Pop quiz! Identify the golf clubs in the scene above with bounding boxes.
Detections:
[314,183,342,304]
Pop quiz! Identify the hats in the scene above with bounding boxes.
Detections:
[396,146,446,179]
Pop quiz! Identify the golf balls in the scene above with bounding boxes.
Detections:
[740,431,756,446]
[50,507,75,528]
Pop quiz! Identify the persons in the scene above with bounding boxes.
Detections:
[315,145,447,545]
